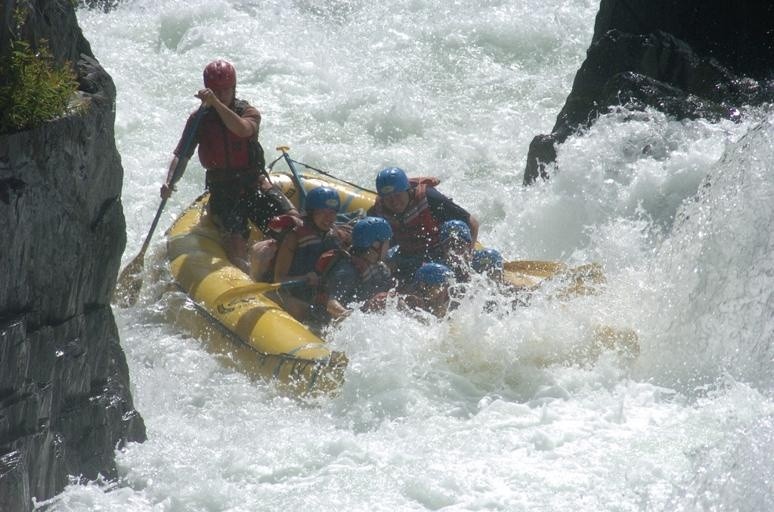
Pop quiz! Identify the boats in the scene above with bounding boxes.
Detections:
[163,169,643,411]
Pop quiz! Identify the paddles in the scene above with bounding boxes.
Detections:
[512,263,608,309]
[212,272,322,305]
[504,260,567,275]
[109,99,209,310]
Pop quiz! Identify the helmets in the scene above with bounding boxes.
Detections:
[413,264,456,290]
[473,248,502,273]
[203,60,236,91]
[376,167,410,198]
[352,216,392,248]
[304,187,340,212]
[439,220,473,248]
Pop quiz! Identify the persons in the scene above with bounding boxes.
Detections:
[274,186,342,286]
[323,218,395,320]
[368,167,479,252]
[470,248,506,278]
[438,220,474,265]
[160,62,298,284]
[414,267,457,311]
